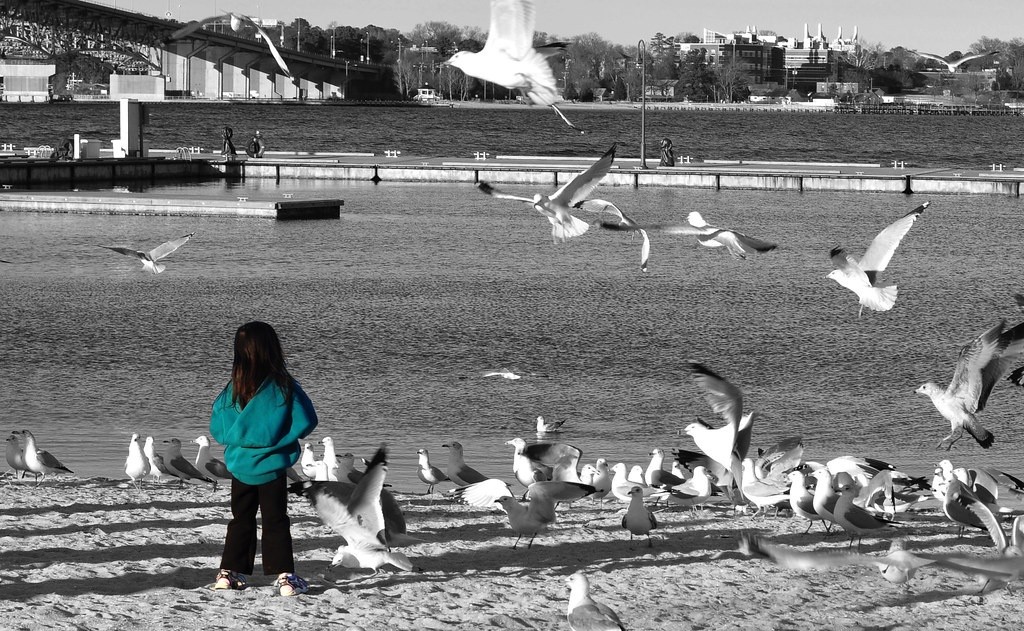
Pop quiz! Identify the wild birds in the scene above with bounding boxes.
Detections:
[558,573,626,631]
[163,438,215,488]
[220,9,291,79]
[102,232,196,274]
[913,318,1024,452]
[12,429,74,487]
[600,211,778,260]
[124,433,171,490]
[295,357,1024,600]
[435,0,586,135]
[478,142,617,245]
[190,436,233,483]
[823,200,931,318]
[573,198,650,272]
[5,435,36,479]
[907,50,999,72]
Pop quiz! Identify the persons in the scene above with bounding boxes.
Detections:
[209,322,319,596]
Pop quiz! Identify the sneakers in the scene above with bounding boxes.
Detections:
[213,569,248,590]
[274,573,310,596]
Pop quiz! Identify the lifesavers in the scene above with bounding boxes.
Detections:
[244,136,265,158]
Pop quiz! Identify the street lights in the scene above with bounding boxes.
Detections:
[295,17,301,52]
[366,31,369,63]
[256,4,262,42]
[330,36,333,58]
[178,4,183,24]
[332,25,337,60]
[633,39,648,169]
